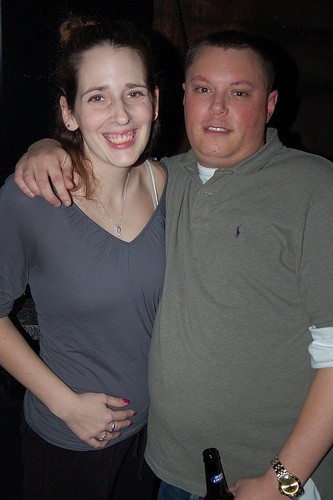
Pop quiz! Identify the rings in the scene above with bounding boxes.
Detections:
[95,430,108,442]
[107,421,116,433]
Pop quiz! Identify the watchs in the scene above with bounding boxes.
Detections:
[270,455,305,500]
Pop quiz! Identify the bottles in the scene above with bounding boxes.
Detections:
[200,446,238,499]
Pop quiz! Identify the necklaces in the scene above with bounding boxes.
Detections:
[90,171,129,234]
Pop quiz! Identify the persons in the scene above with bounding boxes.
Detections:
[15,30,333,500]
[0,18,170,500]
